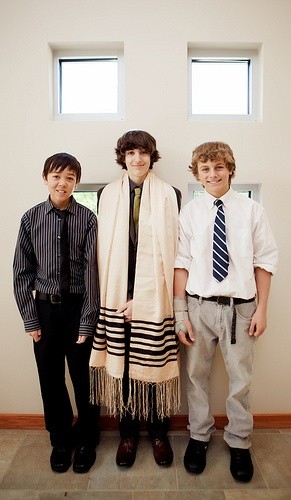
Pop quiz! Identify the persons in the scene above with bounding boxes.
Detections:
[173,142,278,484]
[13,152,100,473]
[89,130,182,468]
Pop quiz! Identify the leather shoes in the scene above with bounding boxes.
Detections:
[229,447,254,482]
[73,445,97,473]
[184,438,208,474]
[150,430,174,465]
[50,441,74,473]
[116,437,138,467]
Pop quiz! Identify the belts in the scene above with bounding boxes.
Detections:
[185,290,257,344]
[38,292,80,304]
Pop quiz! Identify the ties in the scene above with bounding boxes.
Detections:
[212,199,229,282]
[52,207,70,305]
[133,187,142,243]
